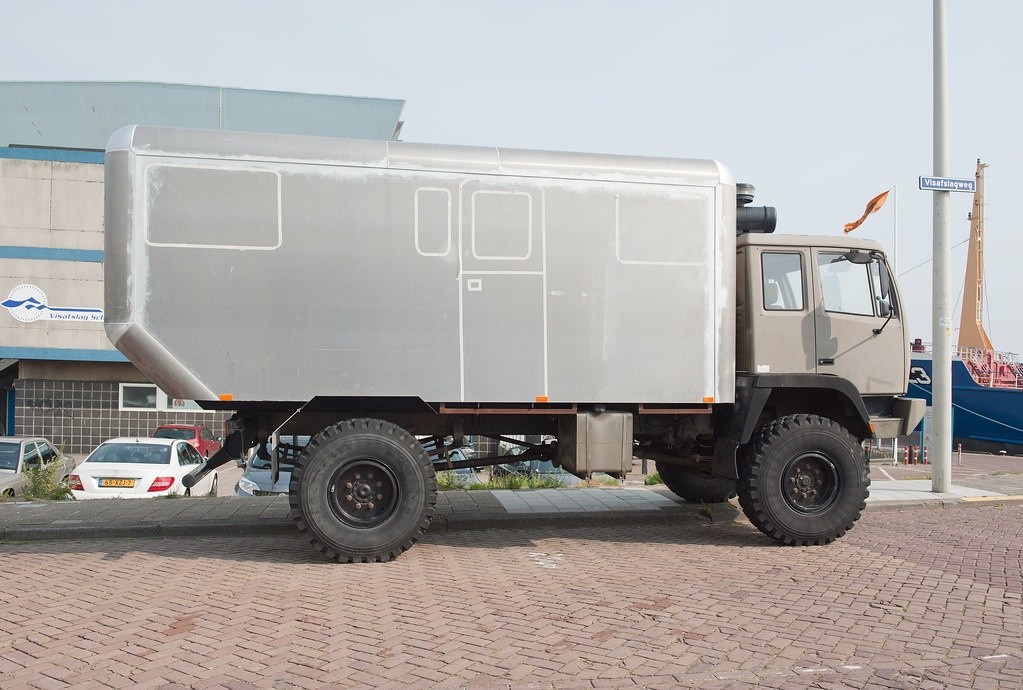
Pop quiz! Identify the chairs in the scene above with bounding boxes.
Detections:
[103,449,164,464]
[764,282,783,309]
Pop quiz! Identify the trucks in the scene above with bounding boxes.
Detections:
[106,124,927,566]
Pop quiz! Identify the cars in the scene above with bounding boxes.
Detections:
[62,424,222,499]
[490,445,581,488]
[235,434,311,497]
[416,440,476,482]
[0,436,77,501]
